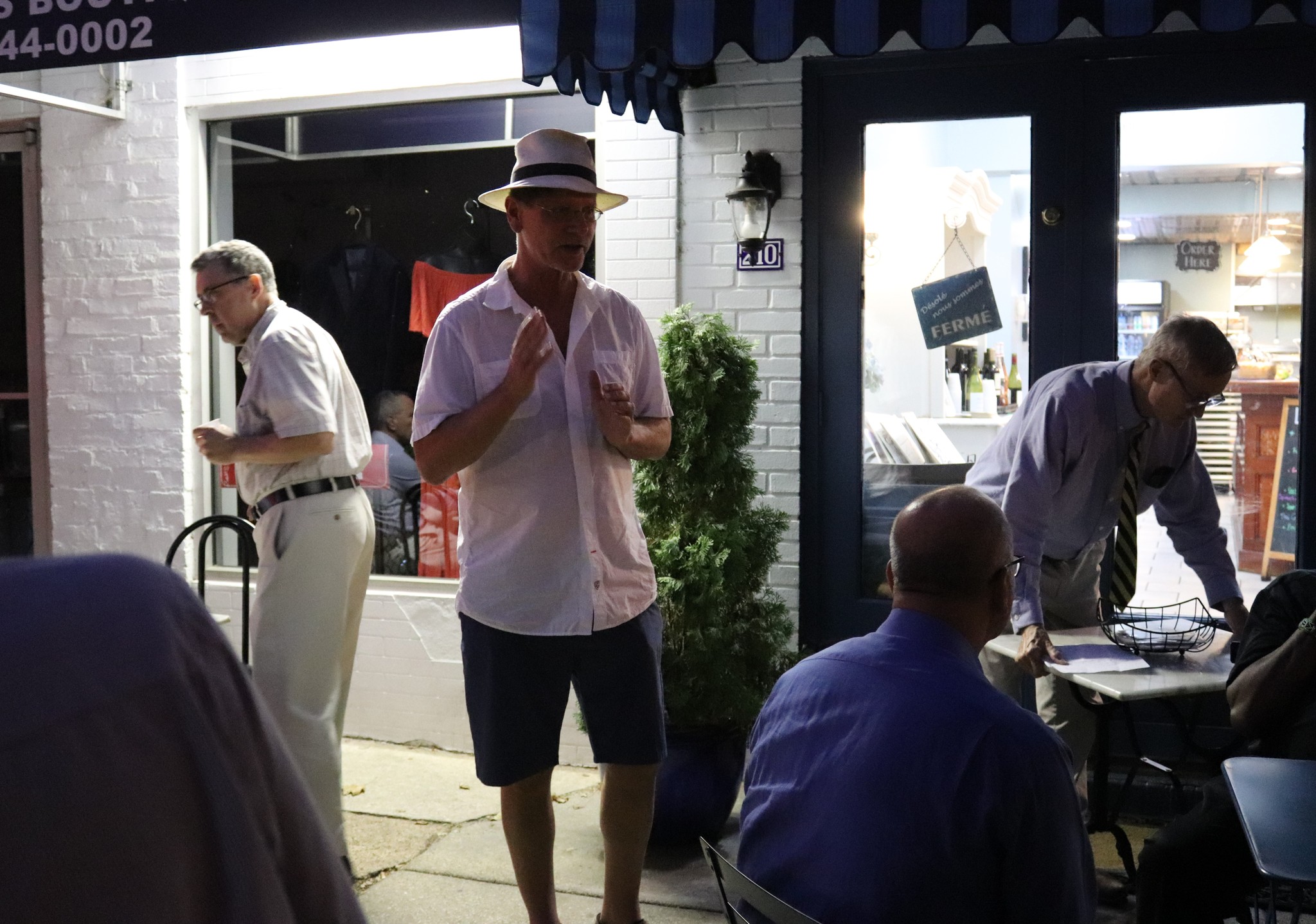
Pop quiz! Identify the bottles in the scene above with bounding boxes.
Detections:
[944,344,1021,415]
[1117,313,1160,358]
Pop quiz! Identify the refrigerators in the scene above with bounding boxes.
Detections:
[1116,280,1170,362]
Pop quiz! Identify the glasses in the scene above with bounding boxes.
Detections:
[194,276,249,312]
[523,199,603,224]
[988,554,1026,594]
[1162,358,1225,409]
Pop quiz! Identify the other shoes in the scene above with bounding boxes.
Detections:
[1094,868,1125,904]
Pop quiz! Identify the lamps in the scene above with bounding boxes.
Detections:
[724,146,783,268]
[1235,163,1291,280]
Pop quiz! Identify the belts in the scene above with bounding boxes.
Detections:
[250,475,360,521]
[1042,553,1077,573]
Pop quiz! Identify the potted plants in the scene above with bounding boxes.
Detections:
[573,309,811,861]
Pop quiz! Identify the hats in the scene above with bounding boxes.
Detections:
[476,127,628,213]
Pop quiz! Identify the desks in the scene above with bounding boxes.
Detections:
[982,617,1236,896]
[1221,757,1316,924]
[917,414,1012,462]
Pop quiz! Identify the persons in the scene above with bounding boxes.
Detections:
[409,130,675,924]
[190,241,377,883]
[358,390,469,579]
[965,312,1316,924]
[732,485,1109,924]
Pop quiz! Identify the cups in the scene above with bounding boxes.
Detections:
[195,418,222,438]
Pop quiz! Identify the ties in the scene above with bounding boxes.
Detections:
[1107,420,1152,615]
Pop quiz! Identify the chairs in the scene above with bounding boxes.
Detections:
[699,836,822,924]
[165,513,255,664]
[399,481,458,577]
